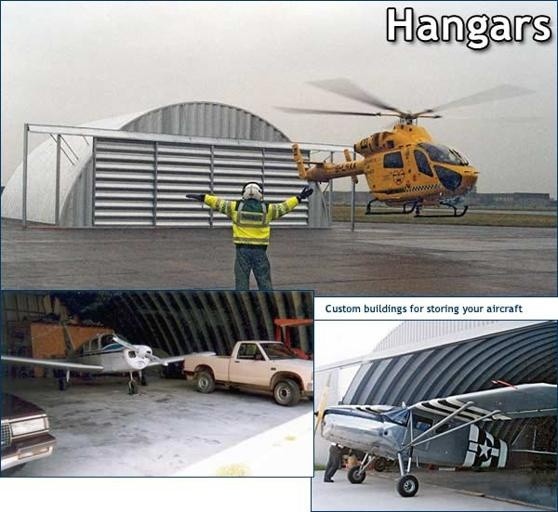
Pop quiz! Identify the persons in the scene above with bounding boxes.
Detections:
[182,181,314,290]
[322,442,345,483]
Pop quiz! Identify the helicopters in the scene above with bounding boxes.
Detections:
[271,78,536,219]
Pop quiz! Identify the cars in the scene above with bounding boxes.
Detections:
[0,392,57,477]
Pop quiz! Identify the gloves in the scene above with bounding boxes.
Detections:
[186,193,204,203]
[297,187,313,202]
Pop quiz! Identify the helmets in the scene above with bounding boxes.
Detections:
[242,182,263,202]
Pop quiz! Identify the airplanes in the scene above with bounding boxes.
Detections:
[313,371,558,497]
[0,321,216,395]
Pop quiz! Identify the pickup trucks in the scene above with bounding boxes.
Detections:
[182,339,314,406]
